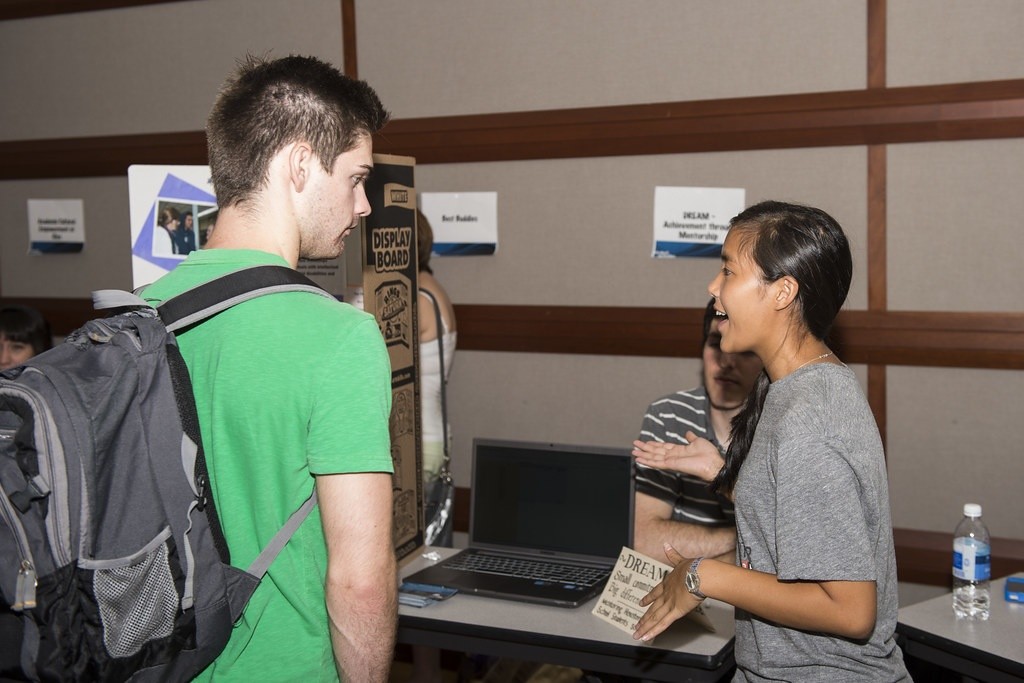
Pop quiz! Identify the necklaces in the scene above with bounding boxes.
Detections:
[791,350,833,373]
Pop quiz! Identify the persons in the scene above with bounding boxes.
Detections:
[128,47,402,682]
[416,207,458,550]
[153,207,215,256]
[1,299,55,373]
[630,198,916,683]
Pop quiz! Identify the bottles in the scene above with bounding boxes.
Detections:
[951,503,991,622]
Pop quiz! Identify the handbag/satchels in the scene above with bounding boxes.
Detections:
[419,469,454,552]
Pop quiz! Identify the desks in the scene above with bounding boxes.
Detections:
[398,546,737,683]
[896,571,1024,683]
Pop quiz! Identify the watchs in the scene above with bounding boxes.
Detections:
[683,556,708,603]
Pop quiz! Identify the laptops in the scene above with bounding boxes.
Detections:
[403,438,636,609]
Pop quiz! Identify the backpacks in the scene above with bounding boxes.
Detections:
[0,263,339,683]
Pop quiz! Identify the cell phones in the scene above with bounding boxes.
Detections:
[1005,577,1024,604]
